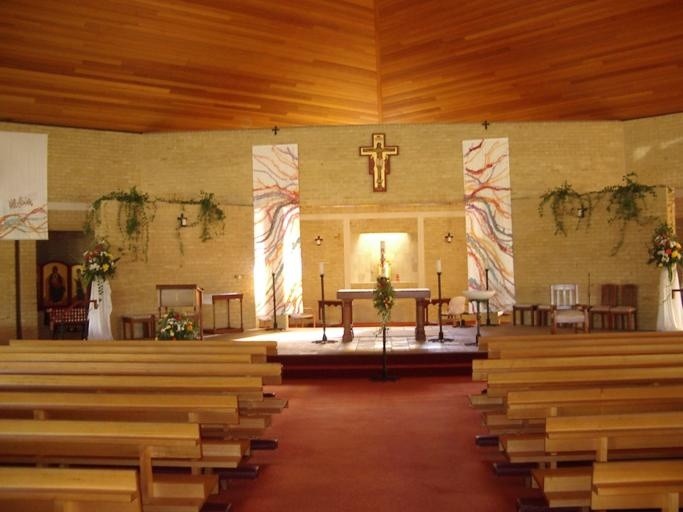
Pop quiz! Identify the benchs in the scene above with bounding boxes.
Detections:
[461,332,681,512]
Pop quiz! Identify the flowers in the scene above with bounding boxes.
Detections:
[75,236,119,296]
[643,221,682,282]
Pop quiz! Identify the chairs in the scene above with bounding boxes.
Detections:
[511,275,639,331]
[116,282,243,337]
[436,258,442,273]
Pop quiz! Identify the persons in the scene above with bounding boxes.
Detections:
[71,267,88,303]
[45,265,67,303]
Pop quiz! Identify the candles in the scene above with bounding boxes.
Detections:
[318,258,323,274]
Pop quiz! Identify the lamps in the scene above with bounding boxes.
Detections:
[315,235,322,245]
[177,211,188,227]
[445,231,453,242]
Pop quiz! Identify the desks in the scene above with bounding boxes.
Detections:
[337,289,432,341]
